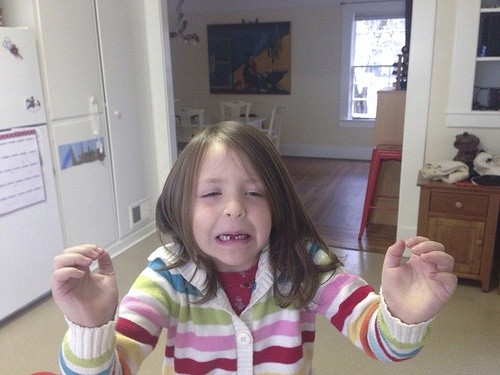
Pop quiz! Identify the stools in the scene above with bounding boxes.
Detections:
[356,144,402,240]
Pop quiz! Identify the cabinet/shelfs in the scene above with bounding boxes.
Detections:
[416,171,500,293]
[369,86,407,225]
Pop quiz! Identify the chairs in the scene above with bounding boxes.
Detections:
[220,101,251,118]
[174,108,204,121]
[257,104,288,156]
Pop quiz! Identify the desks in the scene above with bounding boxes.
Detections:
[175,116,267,136]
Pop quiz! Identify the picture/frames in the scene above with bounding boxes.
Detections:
[207,21,292,95]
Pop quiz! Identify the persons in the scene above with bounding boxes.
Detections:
[50,119,459,375]
